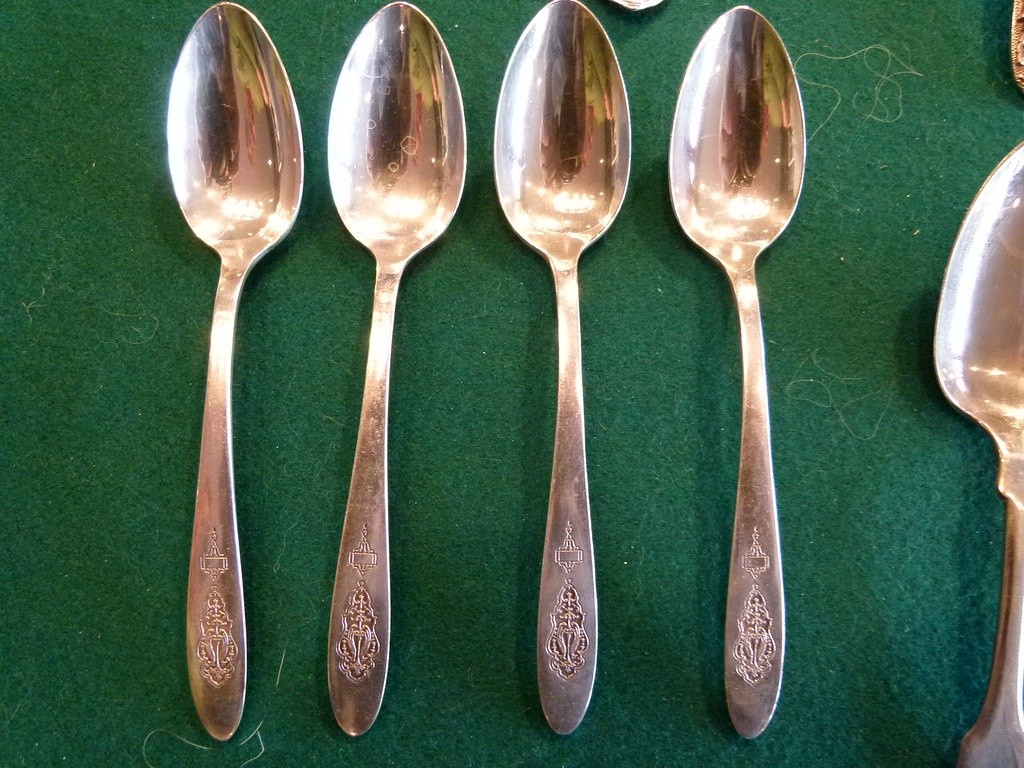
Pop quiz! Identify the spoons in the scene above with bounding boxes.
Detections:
[165,1,303,742]
[493,0,631,736]
[932,138,1024,768]
[326,0,467,737]
[668,5,806,740]
